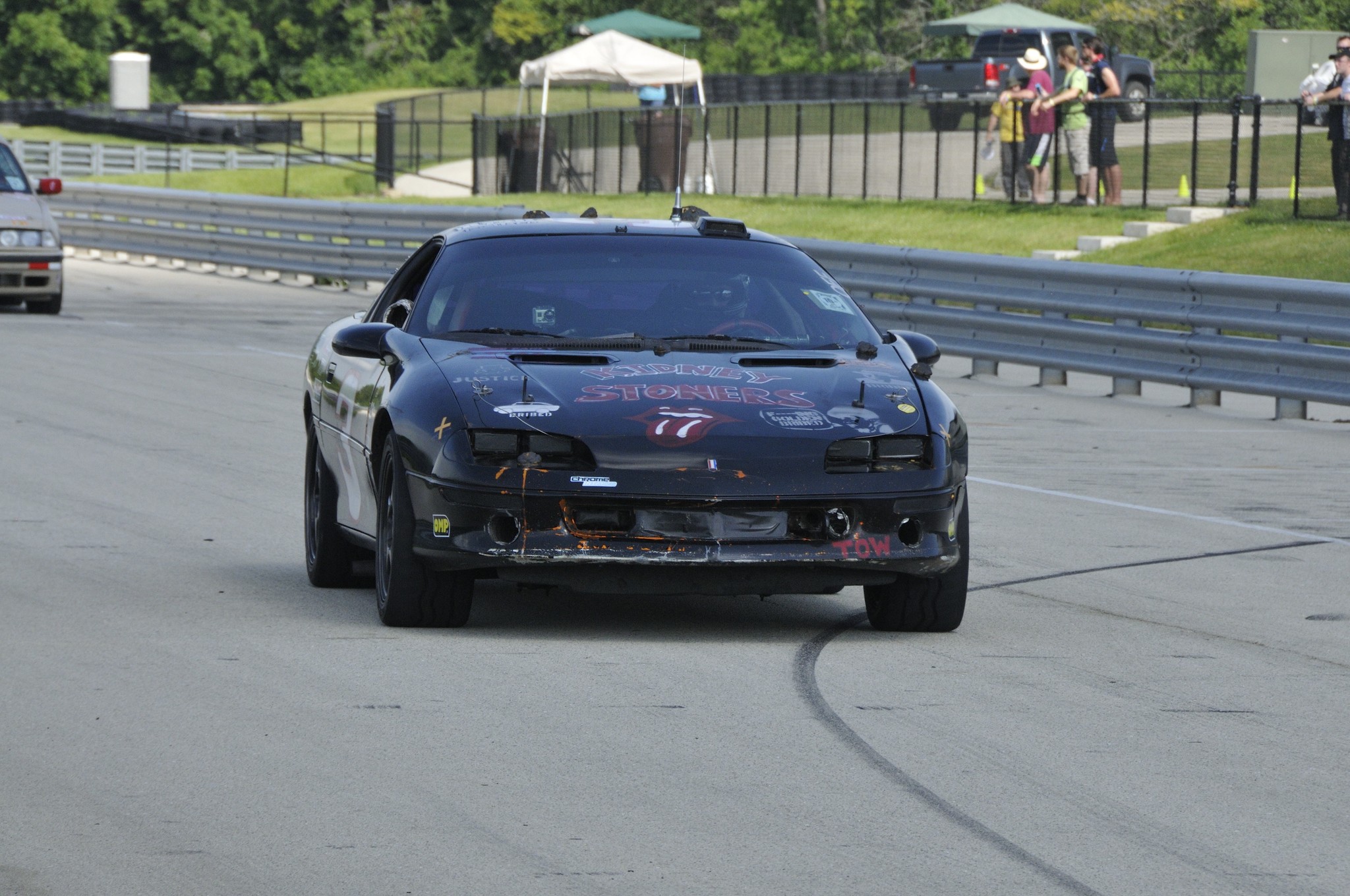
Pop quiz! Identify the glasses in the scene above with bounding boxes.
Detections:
[692,287,732,303]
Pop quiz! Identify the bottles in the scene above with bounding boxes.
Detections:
[1305,94,1316,111]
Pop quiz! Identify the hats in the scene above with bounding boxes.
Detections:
[1005,76,1022,89]
[1017,47,1048,70]
[1328,47,1350,59]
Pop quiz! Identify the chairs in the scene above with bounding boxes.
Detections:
[554,142,592,193]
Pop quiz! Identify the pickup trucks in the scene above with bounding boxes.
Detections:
[907,27,1156,131]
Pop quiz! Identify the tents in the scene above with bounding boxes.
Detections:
[920,0,1101,40]
[505,28,718,197]
[569,7,702,113]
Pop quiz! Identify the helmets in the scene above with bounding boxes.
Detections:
[687,268,752,323]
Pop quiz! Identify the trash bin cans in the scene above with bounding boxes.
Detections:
[636,117,693,192]
[501,127,554,192]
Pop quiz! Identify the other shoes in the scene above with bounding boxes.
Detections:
[1325,210,1350,220]
[1018,190,1029,198]
[1030,199,1043,205]
[1067,198,1087,208]
[1101,200,1121,208]
[1004,196,1014,203]
[1085,196,1097,208]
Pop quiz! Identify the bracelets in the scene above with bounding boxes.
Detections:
[1311,94,1319,105]
[1007,93,1011,99]
[1048,98,1055,107]
[1093,93,1098,101]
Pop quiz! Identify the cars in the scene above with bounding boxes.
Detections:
[302,217,968,632]
[0,138,67,314]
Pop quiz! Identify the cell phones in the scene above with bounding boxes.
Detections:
[1035,84,1041,94]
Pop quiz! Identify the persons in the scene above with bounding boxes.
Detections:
[985,38,1125,207]
[675,267,756,338]
[1300,35,1350,222]
